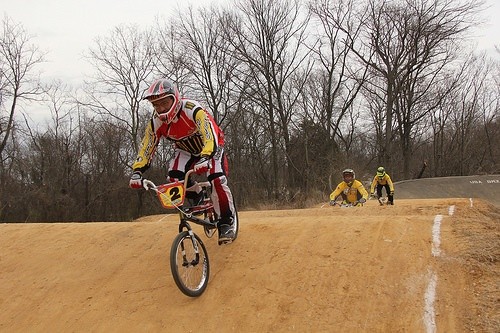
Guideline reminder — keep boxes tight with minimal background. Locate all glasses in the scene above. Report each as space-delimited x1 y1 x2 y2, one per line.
152 98 171 108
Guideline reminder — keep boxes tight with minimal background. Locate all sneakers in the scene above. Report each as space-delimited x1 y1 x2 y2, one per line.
216 216 235 245
192 193 205 216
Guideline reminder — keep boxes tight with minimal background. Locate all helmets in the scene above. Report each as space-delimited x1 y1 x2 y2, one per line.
143 79 181 124
377 167 385 176
342 169 355 180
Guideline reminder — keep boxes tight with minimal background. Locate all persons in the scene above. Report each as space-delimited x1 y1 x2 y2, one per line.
330 168 368 206
129 78 236 242
371 166 394 205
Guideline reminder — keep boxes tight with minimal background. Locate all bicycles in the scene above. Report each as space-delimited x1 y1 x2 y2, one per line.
334 201 363 207
129 162 239 297
370 193 394 206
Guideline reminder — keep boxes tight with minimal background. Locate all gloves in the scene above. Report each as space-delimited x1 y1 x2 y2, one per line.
370 193 375 197
330 200 336 206
359 198 366 203
390 191 394 195
130 171 144 188
194 157 212 175
